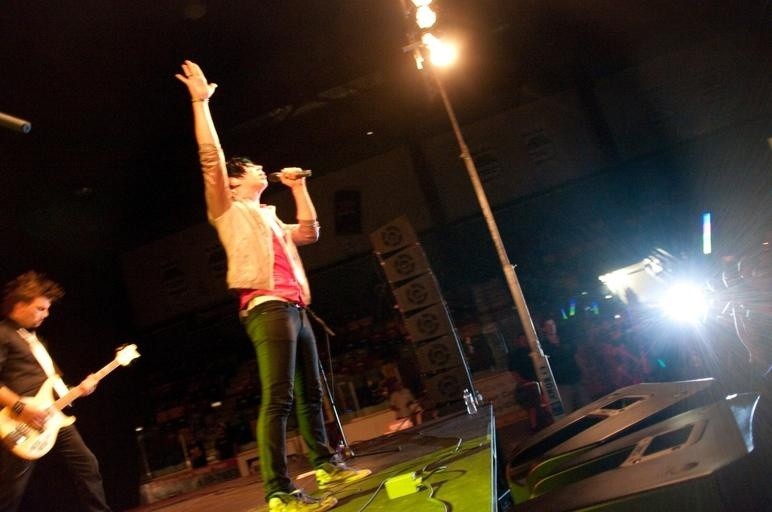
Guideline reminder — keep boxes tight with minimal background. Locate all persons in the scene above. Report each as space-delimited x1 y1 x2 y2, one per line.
176 58 372 511
0 268 118 508
507 238 771 512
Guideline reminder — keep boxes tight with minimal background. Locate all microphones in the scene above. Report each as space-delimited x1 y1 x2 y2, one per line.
267 170 313 183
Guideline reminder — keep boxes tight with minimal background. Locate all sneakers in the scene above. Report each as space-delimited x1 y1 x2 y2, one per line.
315 460 371 490
268 490 338 512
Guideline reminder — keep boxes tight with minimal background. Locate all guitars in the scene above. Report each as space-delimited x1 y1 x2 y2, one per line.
0 343 141 461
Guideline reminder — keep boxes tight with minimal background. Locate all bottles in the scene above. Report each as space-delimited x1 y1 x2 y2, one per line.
463 389 478 415
475 390 485 407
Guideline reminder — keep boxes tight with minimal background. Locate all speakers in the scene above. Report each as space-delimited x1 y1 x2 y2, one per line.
369 216 478 404
505 377 772 512
333 189 361 235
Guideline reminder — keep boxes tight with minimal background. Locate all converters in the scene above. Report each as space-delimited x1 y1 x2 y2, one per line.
385 472 420 500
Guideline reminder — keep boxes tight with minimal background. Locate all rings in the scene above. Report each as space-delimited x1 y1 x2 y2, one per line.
187 73 193 78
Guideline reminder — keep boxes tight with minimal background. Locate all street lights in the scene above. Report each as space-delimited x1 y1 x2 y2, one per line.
401 0 568 419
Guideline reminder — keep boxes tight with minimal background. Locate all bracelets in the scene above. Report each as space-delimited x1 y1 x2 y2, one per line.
12 400 25 416
191 98 210 104
